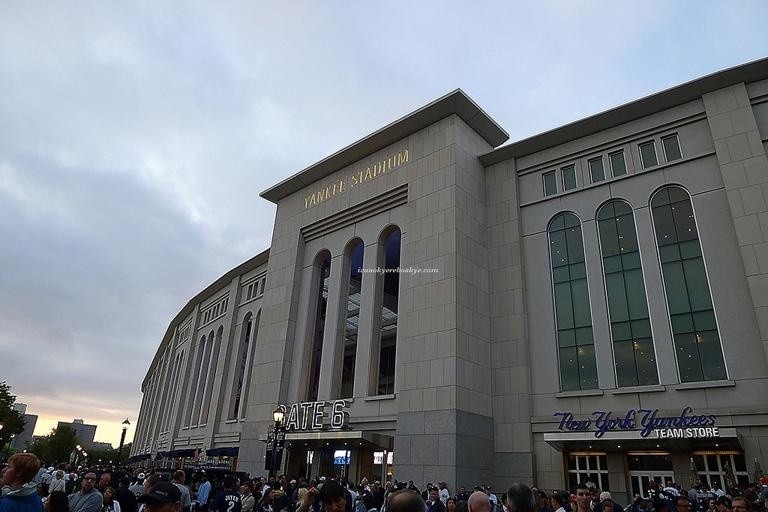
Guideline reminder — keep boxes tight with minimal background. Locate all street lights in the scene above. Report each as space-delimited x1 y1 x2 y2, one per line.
116 419 130 466
5 432 15 461
267 406 284 478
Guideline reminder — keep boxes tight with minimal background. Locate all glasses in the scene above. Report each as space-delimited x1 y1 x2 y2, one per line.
577 490 590 497
84 477 96 481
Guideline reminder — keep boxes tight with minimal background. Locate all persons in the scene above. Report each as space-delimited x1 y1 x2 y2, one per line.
0 447 767 511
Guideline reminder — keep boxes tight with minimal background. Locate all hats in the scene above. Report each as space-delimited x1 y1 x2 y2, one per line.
138 473 145 479
